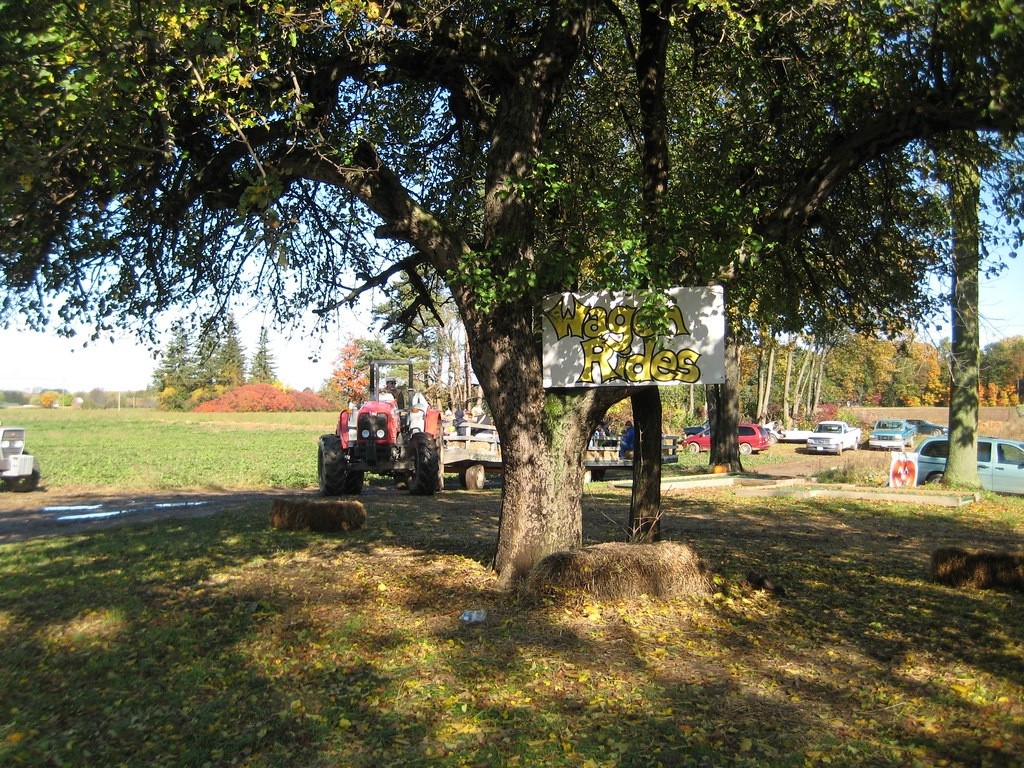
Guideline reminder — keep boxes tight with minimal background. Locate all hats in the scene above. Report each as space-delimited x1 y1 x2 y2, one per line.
386 377 396 382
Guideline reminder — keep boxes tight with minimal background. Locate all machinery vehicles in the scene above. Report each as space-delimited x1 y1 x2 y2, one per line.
0 426 41 494
318 355 680 499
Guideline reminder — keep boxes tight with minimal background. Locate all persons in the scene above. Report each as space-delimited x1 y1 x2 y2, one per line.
378 378 404 410
773 422 786 438
618 420 635 460
439 398 496 453
588 414 616 450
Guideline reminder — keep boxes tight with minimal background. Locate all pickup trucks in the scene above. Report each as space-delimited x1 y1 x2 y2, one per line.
806 420 862 456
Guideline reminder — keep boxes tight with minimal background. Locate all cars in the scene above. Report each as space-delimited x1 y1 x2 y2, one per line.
681 420 813 456
913 435 1024 497
906 419 949 437
869 418 918 452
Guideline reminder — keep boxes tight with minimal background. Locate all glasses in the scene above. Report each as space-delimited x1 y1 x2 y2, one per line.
391 381 396 385
